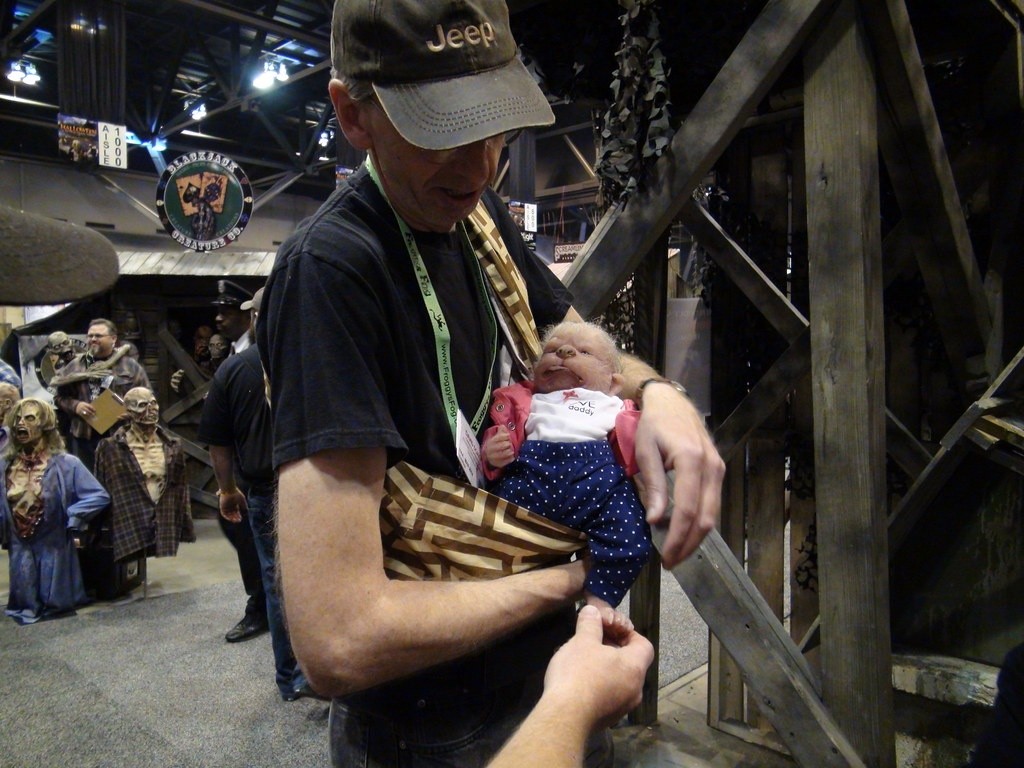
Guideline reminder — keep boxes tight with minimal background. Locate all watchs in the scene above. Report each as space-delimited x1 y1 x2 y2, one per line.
635 378 689 411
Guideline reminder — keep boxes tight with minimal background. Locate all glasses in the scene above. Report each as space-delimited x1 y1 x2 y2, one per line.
88 332 110 338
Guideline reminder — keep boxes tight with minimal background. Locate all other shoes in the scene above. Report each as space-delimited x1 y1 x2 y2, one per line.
281 682 333 701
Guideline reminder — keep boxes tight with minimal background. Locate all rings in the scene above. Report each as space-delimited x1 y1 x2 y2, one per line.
83 411 87 416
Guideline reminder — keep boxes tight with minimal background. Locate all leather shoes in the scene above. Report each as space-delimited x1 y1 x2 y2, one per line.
224 615 270 642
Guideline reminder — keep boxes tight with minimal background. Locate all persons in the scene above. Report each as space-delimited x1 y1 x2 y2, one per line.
261 0 727 767
54 317 153 477
195 285 305 703
483 605 655 768
204 280 270 643
481 321 652 643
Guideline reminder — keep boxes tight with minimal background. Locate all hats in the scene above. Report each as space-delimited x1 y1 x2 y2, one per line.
240 286 268 311
211 279 252 307
329 0 557 151
